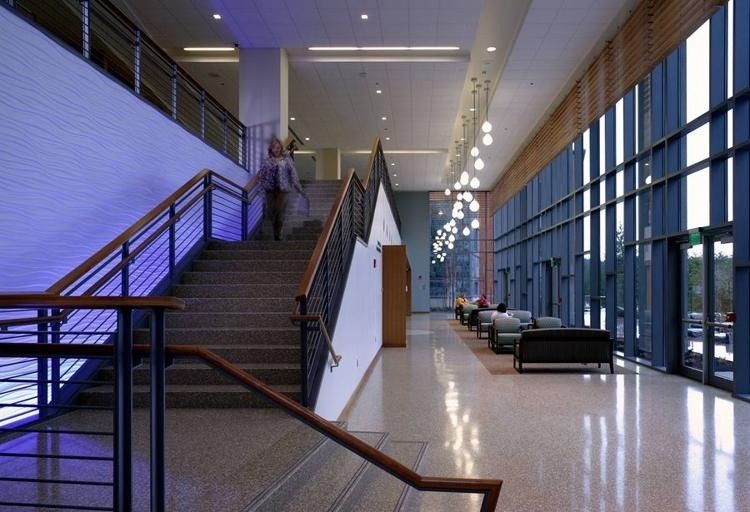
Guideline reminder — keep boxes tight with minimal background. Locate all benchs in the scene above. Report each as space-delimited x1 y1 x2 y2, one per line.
514 328 615 374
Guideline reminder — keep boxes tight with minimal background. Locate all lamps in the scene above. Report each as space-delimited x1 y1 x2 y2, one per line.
432 78 493 265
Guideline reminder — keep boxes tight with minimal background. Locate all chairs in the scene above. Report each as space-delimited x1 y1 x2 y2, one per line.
455 304 569 354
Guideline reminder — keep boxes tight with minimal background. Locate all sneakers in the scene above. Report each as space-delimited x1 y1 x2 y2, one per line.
274 233 283 241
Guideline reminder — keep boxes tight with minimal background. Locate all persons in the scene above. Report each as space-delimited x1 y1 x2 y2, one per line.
474 293 490 308
456 293 468 306
254 138 304 242
491 302 509 329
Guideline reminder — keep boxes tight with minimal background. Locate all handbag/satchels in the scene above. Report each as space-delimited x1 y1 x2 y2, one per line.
295 193 310 216
